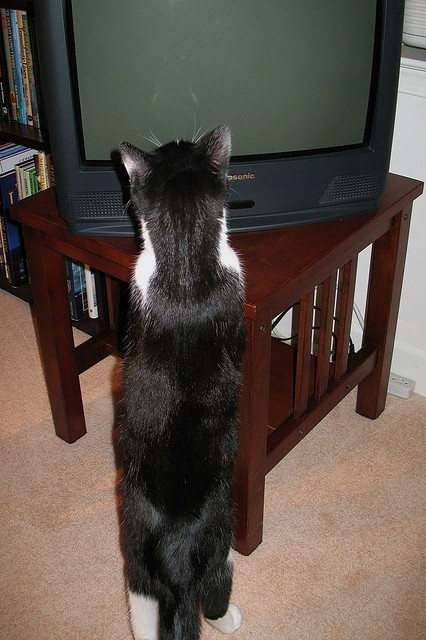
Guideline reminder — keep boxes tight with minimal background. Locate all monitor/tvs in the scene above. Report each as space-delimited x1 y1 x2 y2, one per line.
33 0 405 238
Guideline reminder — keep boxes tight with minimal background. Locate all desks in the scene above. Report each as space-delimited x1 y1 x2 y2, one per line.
7 172 424 556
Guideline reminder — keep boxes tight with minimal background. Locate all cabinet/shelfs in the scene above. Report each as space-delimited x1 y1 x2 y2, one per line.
0 0 106 337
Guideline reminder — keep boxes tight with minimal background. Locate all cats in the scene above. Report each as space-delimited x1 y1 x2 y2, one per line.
112 120 248 635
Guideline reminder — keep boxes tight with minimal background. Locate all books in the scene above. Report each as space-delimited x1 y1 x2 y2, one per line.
0 8 41 128
0 142 103 326
